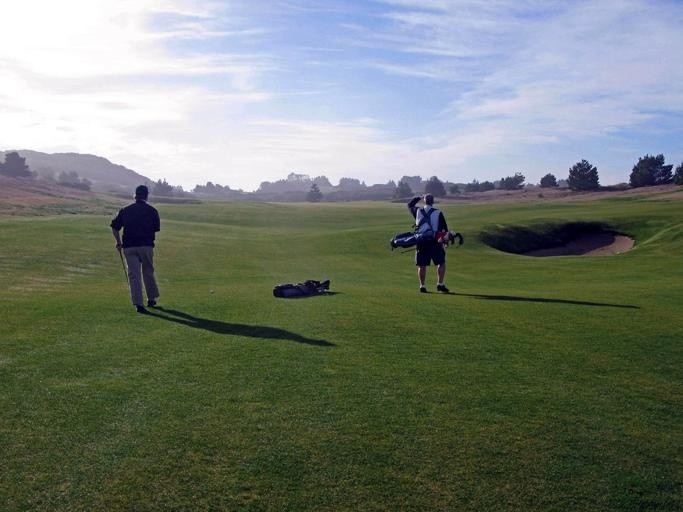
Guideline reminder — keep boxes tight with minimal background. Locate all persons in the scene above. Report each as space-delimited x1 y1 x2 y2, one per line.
406 192 450 293
110 184 160 312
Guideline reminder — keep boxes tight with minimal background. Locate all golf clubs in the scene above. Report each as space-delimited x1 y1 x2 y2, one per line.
120 251 130 289
400 233 464 254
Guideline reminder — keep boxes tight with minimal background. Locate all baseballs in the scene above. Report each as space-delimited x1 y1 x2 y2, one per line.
211 290 215 294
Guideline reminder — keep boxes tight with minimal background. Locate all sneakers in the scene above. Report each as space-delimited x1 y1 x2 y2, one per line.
436 284 448 293
136 305 146 313
146 299 156 306
419 286 427 292
